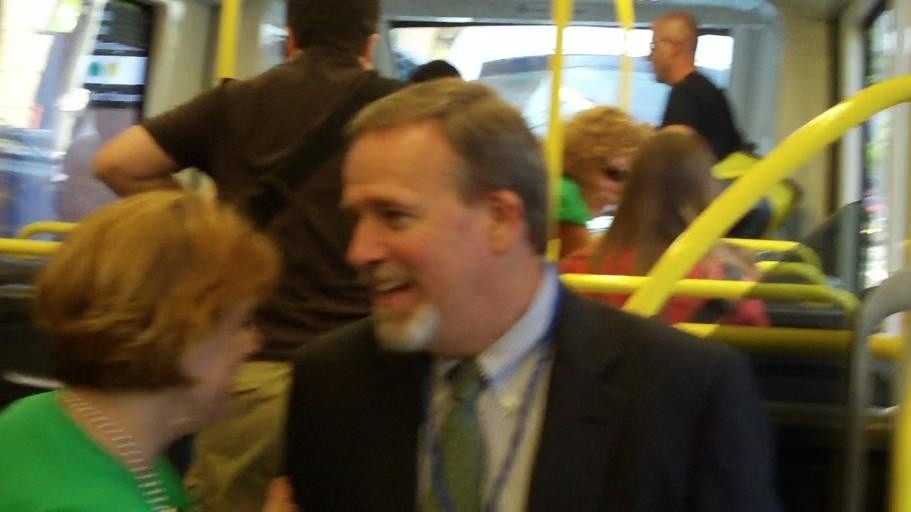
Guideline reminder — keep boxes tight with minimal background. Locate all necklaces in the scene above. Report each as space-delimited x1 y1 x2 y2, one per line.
62 386 171 512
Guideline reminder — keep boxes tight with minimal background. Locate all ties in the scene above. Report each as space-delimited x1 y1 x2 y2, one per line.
431 357 491 510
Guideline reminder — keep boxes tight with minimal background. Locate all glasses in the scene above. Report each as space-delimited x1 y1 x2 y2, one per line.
601 166 629 183
649 38 673 53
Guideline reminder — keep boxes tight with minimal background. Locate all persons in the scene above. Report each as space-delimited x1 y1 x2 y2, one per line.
98 1 420 512
411 59 461 82
556 105 654 257
645 11 771 238
554 124 770 327
0 187 284 512
284 75 776 512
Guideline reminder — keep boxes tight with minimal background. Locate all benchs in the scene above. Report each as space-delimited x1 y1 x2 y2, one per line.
560 238 911 512
0 218 78 391
